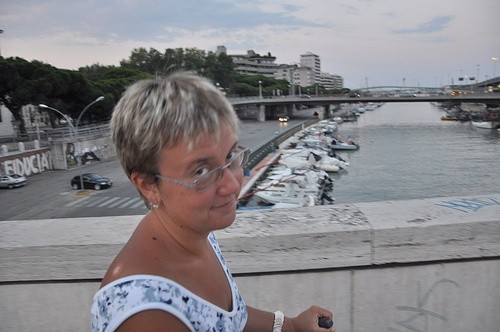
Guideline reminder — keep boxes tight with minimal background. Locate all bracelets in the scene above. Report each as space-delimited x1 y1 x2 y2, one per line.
272 310 284 332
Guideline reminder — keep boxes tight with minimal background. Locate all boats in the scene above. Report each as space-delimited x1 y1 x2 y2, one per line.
254 101 371 210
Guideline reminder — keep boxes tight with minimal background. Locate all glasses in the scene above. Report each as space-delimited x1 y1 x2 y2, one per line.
152 144 252 192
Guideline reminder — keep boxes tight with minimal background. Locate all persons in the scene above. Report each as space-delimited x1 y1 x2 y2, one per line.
89 73 335 332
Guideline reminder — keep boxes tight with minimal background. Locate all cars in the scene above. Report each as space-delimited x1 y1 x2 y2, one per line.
0 173 27 190
71 172 113 191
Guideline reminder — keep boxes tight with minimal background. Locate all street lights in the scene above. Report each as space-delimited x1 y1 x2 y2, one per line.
258 80 263 100
39 96 106 192
